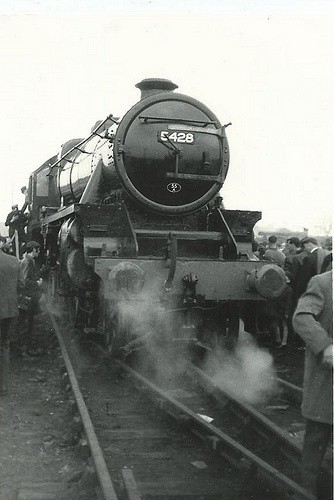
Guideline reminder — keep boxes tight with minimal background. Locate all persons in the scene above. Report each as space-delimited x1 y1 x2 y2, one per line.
252 235 333 491
0 187 45 396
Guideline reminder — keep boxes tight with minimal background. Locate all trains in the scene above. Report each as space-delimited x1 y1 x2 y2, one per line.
4 77 290 359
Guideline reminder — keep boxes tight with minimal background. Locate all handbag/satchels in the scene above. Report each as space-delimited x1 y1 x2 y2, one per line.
17 295 33 313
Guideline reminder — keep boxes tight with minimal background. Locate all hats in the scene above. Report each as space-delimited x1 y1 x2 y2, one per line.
26 240 40 250
300 237 317 246
